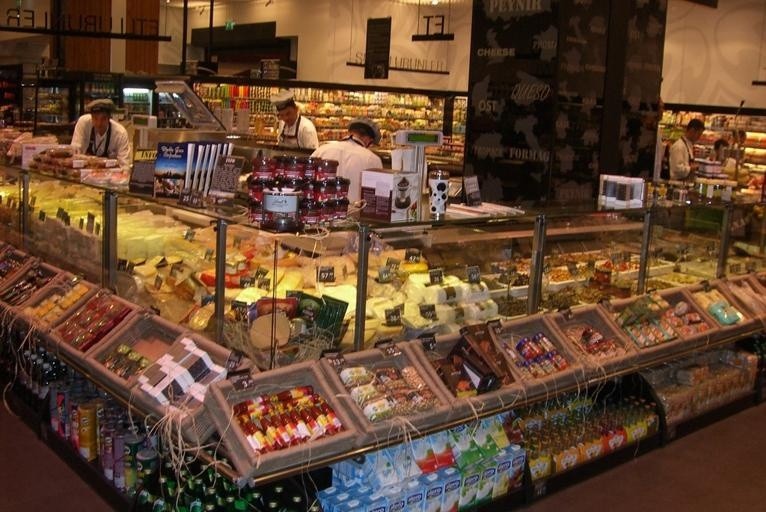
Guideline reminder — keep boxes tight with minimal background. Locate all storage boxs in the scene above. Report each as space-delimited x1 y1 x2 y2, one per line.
361 169 425 224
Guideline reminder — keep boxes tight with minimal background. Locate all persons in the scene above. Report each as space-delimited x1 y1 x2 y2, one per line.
308 114 382 206
269 92 319 150
668 119 705 180
70 99 131 177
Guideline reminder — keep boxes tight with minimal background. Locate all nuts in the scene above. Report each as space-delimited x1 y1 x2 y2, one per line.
377 366 439 415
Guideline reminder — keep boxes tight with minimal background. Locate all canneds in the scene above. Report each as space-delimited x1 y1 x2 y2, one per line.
133 93 148 101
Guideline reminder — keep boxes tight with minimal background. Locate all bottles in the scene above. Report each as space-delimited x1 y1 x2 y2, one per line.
135 449 318 512
524 393 659 482
233 385 344 453
15 346 67 429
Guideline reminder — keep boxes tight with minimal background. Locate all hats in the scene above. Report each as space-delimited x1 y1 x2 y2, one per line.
269 91 294 109
86 98 113 113
349 116 381 145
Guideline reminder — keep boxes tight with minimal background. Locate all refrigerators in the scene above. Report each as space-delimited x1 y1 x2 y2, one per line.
72 69 194 130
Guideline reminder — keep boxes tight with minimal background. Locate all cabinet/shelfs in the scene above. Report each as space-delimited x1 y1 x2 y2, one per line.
200 84 468 171
21 77 193 141
0 161 766 512
659 103 766 203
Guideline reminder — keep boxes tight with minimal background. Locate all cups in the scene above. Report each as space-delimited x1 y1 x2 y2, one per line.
429 170 449 219
